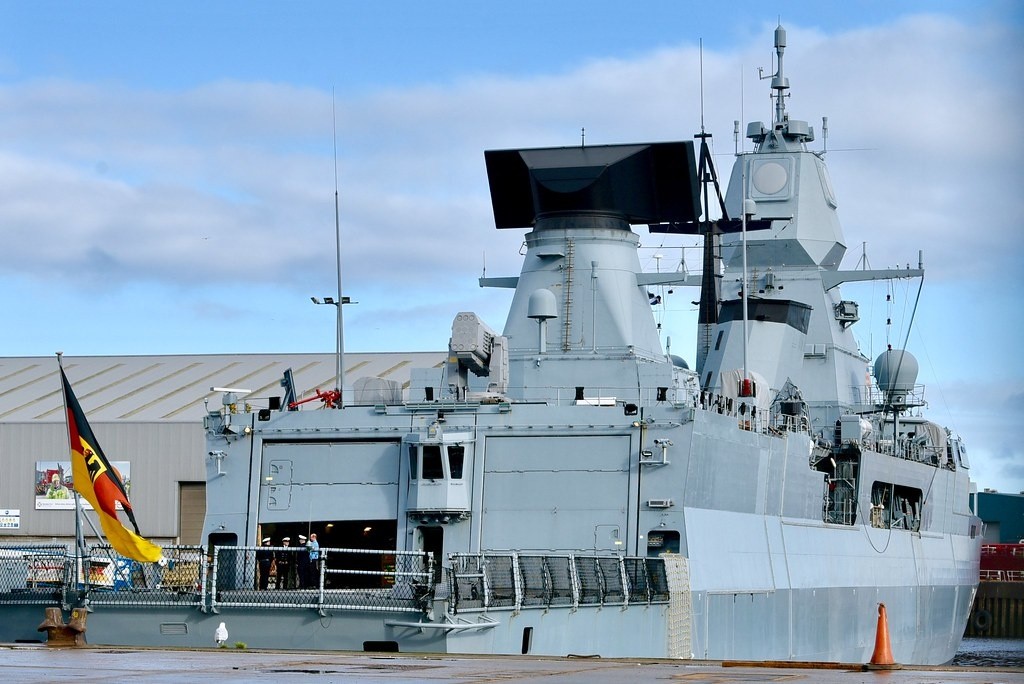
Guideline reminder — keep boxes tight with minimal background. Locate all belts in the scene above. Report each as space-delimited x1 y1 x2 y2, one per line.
259 558 271 562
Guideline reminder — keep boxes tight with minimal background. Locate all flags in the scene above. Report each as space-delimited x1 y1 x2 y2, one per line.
59 365 161 562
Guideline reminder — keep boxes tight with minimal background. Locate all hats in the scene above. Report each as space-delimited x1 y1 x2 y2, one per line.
51 474 60 483
282 537 290 542
261 537 271 543
297 535 307 540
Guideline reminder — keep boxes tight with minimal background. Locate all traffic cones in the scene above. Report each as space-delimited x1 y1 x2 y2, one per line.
863 604 903 669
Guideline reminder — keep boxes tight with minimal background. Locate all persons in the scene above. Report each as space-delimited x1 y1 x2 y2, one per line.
274 537 292 591
257 537 273 590
308 533 320 586
46 474 70 499
296 534 310 589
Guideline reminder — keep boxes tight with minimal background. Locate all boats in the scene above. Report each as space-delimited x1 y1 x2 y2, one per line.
0 15 985 665
961 488 1024 640
